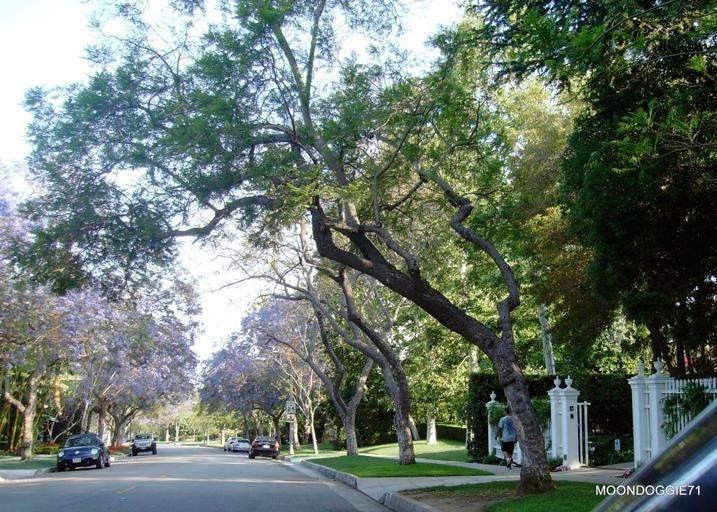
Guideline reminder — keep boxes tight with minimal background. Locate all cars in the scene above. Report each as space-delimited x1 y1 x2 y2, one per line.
249 436 280 459
223 436 250 453
57 433 110 471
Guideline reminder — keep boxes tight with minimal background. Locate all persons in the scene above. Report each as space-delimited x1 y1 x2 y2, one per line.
495 407 517 470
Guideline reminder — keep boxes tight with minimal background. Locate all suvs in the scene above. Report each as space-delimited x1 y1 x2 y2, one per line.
132 433 157 456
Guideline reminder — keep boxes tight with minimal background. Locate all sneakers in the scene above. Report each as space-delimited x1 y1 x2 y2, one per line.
506 457 513 469
